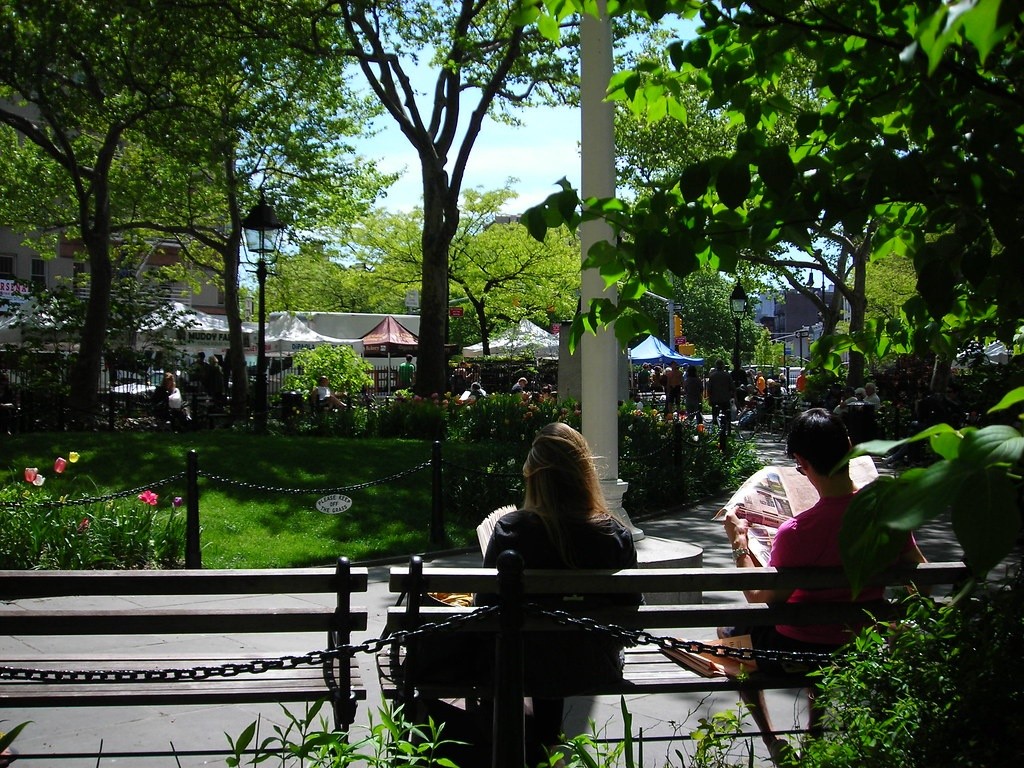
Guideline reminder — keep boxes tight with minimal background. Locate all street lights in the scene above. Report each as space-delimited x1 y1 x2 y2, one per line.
238 190 288 438
806 258 827 306
728 278 749 369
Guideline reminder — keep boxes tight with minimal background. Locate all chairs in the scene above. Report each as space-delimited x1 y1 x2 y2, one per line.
308 393 351 415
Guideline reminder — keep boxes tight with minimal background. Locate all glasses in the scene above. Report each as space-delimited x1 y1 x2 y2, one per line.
796 462 806 476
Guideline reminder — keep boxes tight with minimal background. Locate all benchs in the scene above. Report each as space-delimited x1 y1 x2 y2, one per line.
179 392 229 417
743 392 810 430
373 558 972 768
0 552 369 768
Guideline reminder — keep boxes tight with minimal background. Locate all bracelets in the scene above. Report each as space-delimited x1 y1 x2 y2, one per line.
733 548 751 558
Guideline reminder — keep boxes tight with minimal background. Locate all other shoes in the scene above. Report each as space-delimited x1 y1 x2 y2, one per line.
771 740 801 768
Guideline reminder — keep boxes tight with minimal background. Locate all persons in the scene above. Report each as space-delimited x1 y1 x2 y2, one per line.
157 353 992 768
475 424 641 768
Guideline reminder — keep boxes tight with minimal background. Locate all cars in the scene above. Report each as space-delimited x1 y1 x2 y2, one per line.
110 368 188 397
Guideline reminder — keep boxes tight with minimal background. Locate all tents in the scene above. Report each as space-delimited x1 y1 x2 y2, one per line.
0 301 704 396
358 315 419 395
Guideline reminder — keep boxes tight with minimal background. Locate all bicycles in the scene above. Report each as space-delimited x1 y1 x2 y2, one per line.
737 392 789 444
